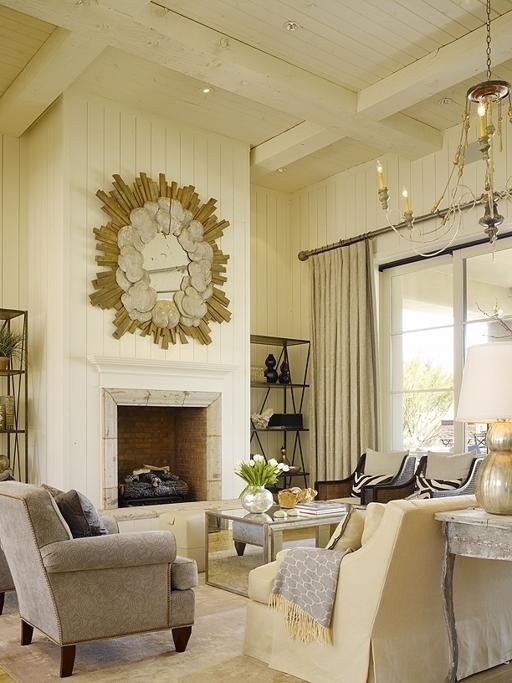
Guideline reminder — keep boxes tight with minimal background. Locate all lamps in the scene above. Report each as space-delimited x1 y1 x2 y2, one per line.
375 0 512 258
456 341 511 516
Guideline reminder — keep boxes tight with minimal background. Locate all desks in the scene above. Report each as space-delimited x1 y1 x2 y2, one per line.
433 508 511 682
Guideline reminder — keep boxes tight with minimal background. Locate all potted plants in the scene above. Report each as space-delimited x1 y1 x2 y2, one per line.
1 321 31 371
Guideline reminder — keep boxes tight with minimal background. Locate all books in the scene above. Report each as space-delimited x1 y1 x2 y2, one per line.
298 506 347 514
295 501 345 510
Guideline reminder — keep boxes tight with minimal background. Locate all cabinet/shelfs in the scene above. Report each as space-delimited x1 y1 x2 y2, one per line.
250 334 311 492
0 308 27 482
